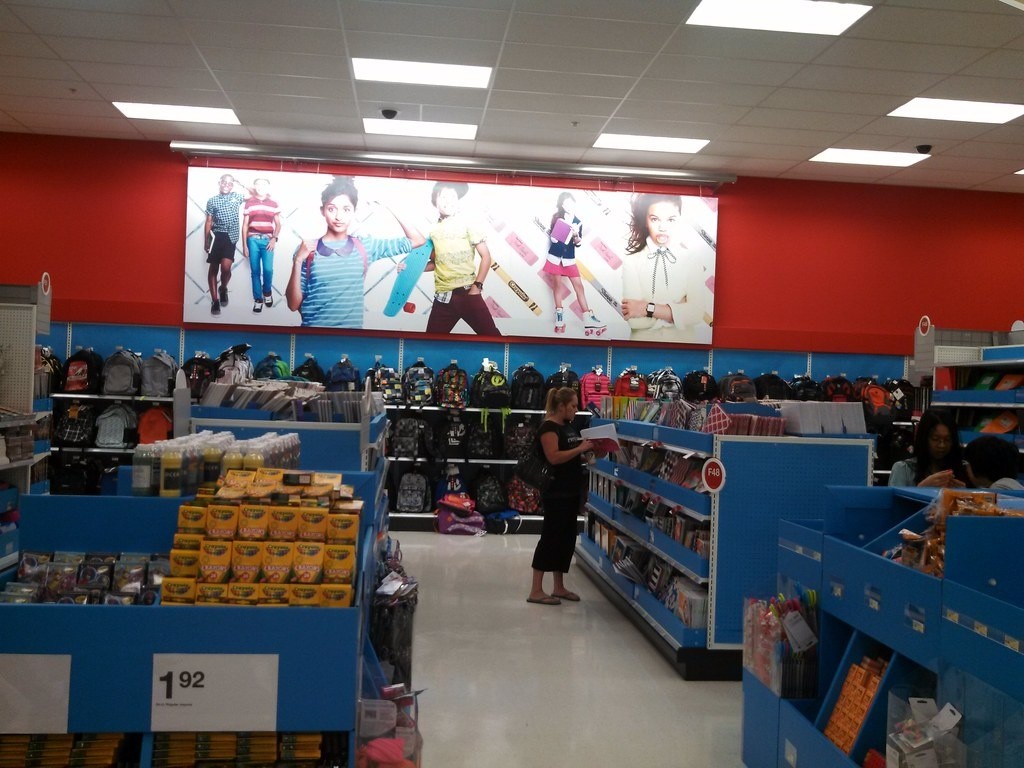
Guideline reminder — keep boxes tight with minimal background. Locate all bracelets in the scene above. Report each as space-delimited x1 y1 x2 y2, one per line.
270 236 278 242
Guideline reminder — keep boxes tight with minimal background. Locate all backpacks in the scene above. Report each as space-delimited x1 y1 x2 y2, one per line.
34 344 915 536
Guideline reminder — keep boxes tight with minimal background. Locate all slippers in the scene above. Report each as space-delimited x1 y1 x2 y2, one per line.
527 595 561 604
551 593 580 601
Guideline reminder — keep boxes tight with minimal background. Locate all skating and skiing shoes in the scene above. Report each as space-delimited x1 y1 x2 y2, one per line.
554 307 566 333
583 309 607 336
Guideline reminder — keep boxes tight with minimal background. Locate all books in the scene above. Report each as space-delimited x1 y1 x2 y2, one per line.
579 423 622 453
206 230 215 254
584 394 788 628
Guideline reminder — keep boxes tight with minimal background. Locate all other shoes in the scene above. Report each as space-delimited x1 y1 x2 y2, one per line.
264 291 272 307
253 299 263 312
218 287 230 307
211 300 221 318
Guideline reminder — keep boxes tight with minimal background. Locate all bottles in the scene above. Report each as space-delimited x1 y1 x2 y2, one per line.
128 429 301 499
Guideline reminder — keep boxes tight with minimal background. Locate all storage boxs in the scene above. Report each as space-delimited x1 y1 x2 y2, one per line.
592 472 644 567
162 468 365 608
0 733 322 768
824 656 889 753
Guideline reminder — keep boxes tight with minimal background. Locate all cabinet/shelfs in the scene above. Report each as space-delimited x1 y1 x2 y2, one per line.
930 344 1024 453
0 419 31 591
741 486 1024 768
0 468 380 768
575 418 877 679
31 412 53 466
48 393 594 521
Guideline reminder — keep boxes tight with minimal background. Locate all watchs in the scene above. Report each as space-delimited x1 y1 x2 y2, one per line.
474 281 484 289
646 302 655 318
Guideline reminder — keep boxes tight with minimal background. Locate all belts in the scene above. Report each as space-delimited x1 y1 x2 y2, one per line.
253 234 272 240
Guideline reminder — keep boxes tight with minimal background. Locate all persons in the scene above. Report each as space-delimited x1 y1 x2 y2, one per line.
242 174 282 313
543 191 608 329
887 408 980 490
526 385 608 607
965 435 1024 491
286 175 427 330
204 174 256 315
620 193 705 344
397 180 502 337
